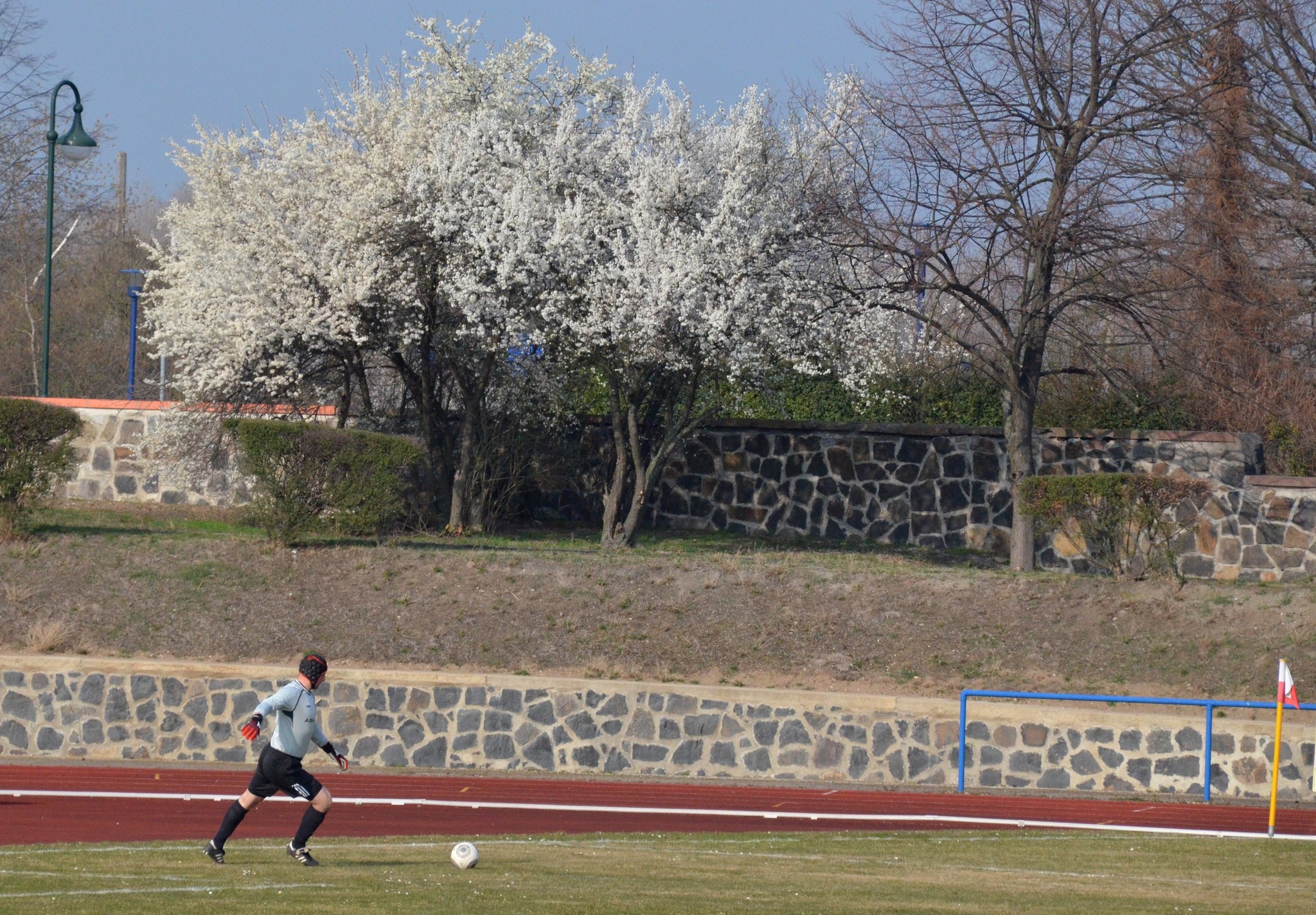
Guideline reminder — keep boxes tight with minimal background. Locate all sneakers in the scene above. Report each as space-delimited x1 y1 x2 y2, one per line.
287 838 319 866
201 839 224 863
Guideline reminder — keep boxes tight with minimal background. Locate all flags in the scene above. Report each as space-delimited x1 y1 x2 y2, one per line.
1276 661 1300 709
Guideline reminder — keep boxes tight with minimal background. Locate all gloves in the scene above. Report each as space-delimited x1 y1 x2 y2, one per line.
240 713 263 741
323 745 348 771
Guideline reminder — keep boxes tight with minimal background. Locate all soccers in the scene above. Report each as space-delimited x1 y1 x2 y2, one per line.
450 842 478 870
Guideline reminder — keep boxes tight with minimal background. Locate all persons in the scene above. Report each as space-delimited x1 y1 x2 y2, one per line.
203 654 349 866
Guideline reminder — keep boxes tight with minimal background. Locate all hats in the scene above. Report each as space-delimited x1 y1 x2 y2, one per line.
298 654 328 685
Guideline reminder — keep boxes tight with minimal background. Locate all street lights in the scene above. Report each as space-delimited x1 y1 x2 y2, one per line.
117 266 148 398
912 220 936 347
41 79 98 400
